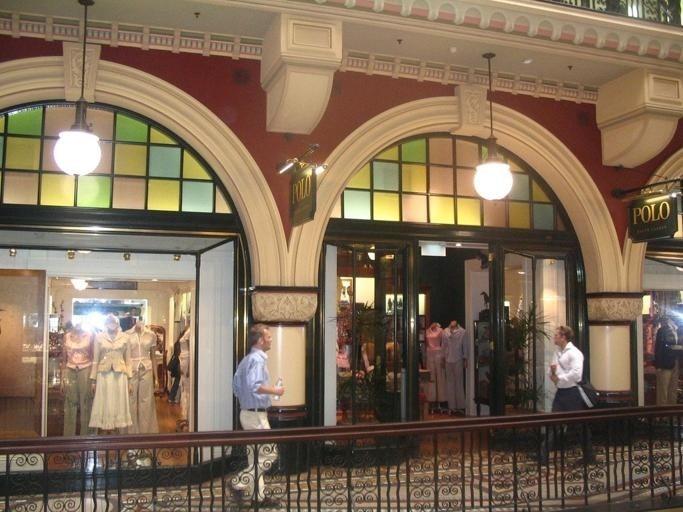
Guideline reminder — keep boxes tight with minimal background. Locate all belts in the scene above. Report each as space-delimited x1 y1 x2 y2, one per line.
557 385 578 391
240 406 268 414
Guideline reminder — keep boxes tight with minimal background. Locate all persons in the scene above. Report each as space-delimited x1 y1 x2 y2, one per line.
655 318 683 423
527 326 596 466
60 310 190 465
441 320 468 410
425 323 443 402
227 323 285 508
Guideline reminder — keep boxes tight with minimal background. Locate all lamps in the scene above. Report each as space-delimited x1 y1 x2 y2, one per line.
174 254 182 260
9 249 16 255
68 250 75 259
474 53 514 201
50 1 102 178
124 254 130 260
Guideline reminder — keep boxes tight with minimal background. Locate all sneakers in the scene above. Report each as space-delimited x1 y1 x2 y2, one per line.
249 496 281 507
577 451 595 465
167 399 179 403
227 480 251 507
525 451 549 466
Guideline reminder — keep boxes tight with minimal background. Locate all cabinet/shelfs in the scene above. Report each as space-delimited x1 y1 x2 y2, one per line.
32 352 81 437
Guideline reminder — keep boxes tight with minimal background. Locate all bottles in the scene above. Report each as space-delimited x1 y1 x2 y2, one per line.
272 377 284 401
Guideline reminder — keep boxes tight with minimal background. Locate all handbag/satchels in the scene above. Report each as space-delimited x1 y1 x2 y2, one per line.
166 355 179 377
577 376 599 409
